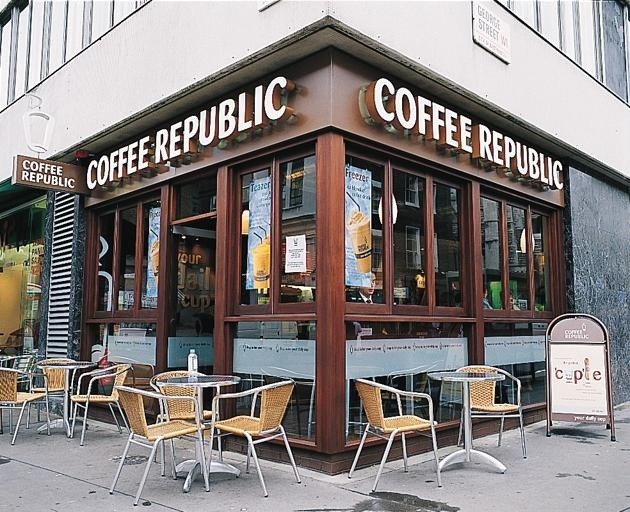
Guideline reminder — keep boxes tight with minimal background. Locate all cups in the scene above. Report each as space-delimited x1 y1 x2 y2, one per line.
346 218 372 273
251 248 270 288
150 248 159 285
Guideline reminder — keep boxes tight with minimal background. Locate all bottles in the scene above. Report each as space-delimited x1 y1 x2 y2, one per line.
187 348 198 378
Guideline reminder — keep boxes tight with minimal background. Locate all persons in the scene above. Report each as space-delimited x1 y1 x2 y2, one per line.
499 289 520 311
346 271 391 335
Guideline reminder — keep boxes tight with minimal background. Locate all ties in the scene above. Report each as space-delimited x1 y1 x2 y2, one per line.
367 299 372 304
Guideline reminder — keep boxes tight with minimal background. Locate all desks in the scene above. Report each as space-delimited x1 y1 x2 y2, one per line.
36 362 95 438
158 375 241 493
428 372 506 473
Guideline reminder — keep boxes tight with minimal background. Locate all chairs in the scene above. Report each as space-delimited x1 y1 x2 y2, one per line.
69 363 130 445
455 365 528 460
110 385 210 505
349 378 443 491
12 355 34 393
151 370 209 481
37 358 75 429
0 368 50 445
206 378 302 497
